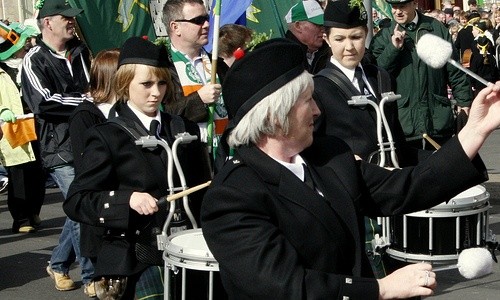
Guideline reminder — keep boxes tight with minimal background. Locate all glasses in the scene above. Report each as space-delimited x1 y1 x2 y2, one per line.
175 14 209 25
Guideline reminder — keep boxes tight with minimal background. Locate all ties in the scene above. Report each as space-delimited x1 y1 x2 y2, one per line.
302 163 315 191
354 67 372 95
148 120 161 140
405 22 416 31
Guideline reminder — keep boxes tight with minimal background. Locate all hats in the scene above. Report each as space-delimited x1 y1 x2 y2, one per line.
473 21 486 32
117 37 169 70
34 0 84 19
9 22 36 37
478 36 489 64
465 13 480 23
444 8 454 14
0 21 27 60
324 0 367 29
222 38 306 129
285 0 324 25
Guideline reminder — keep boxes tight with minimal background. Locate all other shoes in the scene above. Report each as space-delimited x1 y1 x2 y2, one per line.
19 226 36 233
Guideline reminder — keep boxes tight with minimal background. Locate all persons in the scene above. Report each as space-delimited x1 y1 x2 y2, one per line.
61 37 212 300
323 0 437 280
161 0 234 163
21 0 97 297
369 0 473 138
284 0 333 75
431 0 500 95
0 22 48 232
218 24 252 60
69 50 121 257
198 38 500 300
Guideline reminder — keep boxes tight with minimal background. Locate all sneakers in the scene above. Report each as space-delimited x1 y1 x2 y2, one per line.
84 282 96 297
46 265 76 291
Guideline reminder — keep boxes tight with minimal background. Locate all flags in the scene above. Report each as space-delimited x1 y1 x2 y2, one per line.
69 0 299 54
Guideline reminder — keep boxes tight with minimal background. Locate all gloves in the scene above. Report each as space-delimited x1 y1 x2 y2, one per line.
0 108 16 123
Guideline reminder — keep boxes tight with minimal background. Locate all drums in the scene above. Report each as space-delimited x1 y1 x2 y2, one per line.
375 179 491 267
160 228 231 300
